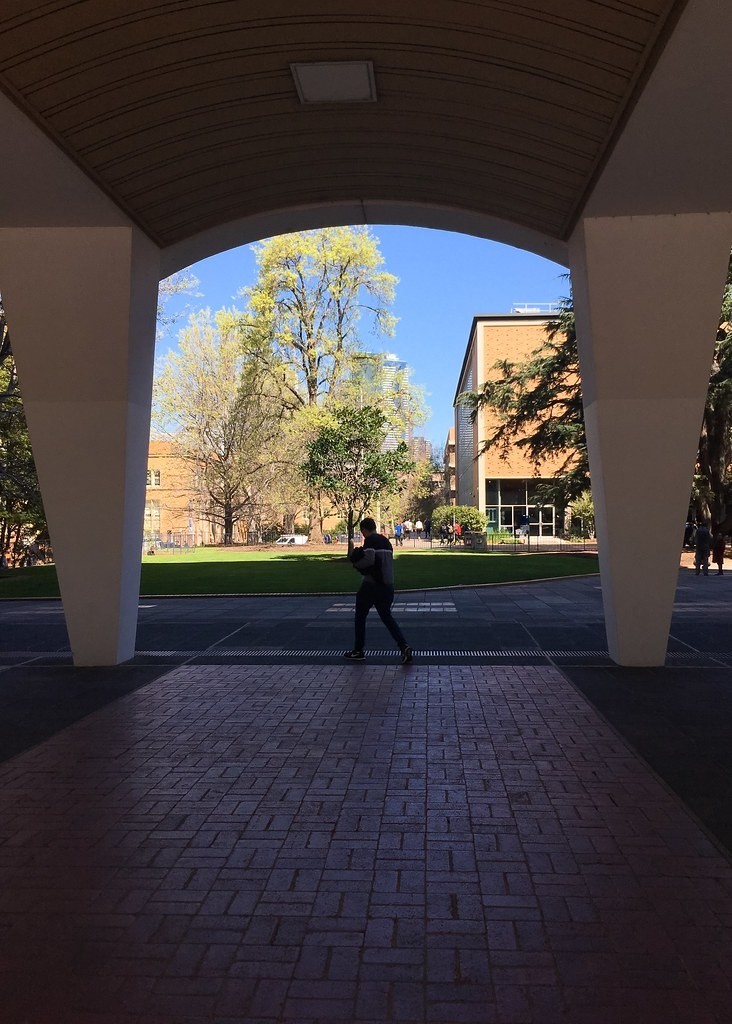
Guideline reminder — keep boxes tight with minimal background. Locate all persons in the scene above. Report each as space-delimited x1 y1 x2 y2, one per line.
343 518 413 664
402 518 431 540
183 541 188 548
712 534 725 575
395 522 403 546
682 521 732 553
438 520 469 545
695 521 711 576
323 530 342 544
355 530 365 545
175 541 181 548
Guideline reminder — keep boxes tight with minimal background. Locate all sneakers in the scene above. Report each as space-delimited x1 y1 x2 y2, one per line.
400 644 412 664
344 651 365 660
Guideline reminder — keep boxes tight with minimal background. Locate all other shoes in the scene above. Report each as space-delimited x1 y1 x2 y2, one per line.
714 570 723 575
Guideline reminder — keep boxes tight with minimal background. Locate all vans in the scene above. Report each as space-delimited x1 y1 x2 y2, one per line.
278 534 308 546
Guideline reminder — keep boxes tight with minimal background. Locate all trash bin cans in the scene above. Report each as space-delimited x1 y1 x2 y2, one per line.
463 530 487 549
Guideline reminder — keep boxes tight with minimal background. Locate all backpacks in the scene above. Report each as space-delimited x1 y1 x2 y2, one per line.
447 525 453 533
